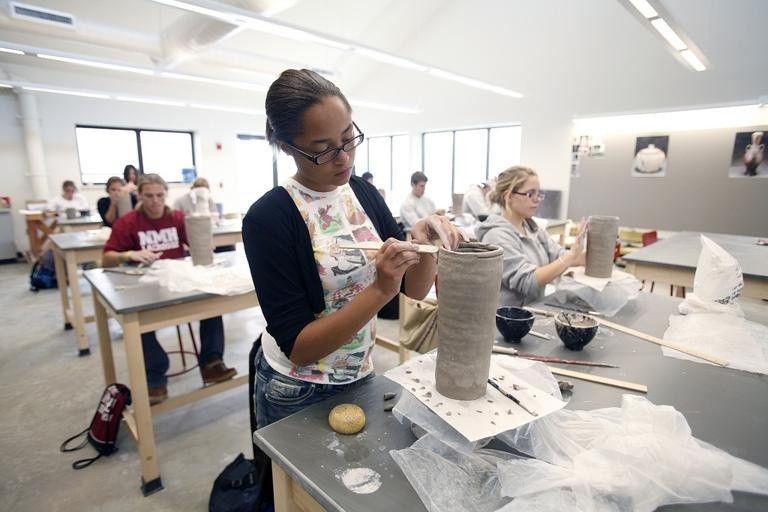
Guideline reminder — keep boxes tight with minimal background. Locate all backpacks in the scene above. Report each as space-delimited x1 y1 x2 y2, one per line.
28 249 60 294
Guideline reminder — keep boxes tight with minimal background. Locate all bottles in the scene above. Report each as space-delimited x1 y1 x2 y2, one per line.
635 143 665 173
743 132 765 176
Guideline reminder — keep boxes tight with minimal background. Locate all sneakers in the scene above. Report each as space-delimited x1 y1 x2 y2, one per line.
199 361 237 386
147 385 171 406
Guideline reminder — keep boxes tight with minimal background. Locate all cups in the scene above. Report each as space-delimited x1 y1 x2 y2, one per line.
584 213 620 280
451 194 465 217
184 214 218 268
434 241 501 402
115 189 131 219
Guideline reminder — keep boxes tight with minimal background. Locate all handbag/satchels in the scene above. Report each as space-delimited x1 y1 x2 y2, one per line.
60 383 134 471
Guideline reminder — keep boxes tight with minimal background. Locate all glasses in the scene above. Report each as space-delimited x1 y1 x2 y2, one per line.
511 190 547 202
280 118 366 166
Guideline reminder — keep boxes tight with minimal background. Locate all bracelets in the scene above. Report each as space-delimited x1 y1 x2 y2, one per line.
558 255 570 269
116 251 133 263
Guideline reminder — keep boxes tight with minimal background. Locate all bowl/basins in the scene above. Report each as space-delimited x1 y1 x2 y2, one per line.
554 310 600 352
496 305 536 345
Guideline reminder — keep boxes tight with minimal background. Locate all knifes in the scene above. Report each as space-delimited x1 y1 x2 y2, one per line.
340 242 438 254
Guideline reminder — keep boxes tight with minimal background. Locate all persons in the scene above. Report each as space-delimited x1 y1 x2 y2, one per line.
173 176 235 255
244 66 472 512
103 173 238 407
123 166 140 183
363 172 376 187
473 165 588 313
97 176 138 230
400 167 445 235
43 182 97 271
